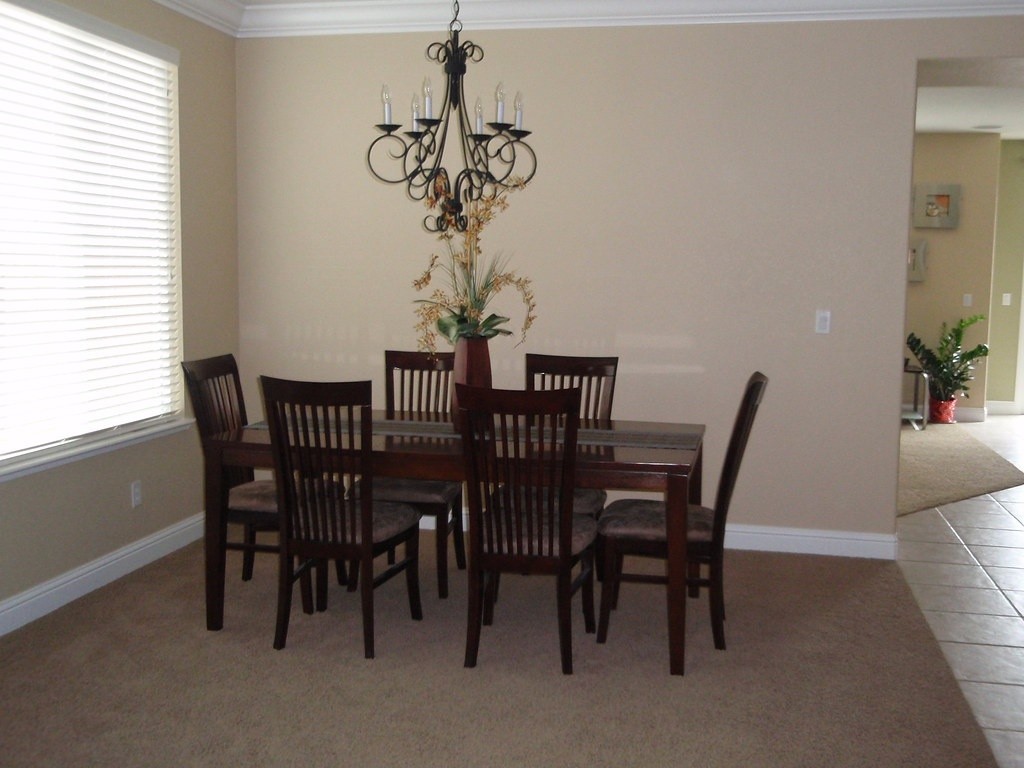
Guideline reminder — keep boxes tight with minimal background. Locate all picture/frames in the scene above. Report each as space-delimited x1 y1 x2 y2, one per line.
912 182 962 229
908 239 928 281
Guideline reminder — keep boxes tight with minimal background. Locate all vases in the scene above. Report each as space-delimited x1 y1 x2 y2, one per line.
452 336 492 418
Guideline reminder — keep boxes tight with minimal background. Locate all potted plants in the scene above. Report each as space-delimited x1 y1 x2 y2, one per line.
907 316 989 424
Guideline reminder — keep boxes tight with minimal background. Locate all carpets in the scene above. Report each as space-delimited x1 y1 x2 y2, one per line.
897 424 1024 518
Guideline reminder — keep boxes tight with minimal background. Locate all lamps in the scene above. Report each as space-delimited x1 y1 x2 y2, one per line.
367 0 537 231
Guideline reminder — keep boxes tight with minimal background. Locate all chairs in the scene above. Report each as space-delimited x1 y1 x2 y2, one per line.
595 370 768 649
347 351 466 597
485 353 619 580
181 355 347 615
256 376 423 659
456 383 596 676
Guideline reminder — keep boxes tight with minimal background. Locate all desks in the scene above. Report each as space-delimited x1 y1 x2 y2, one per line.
901 365 929 432
212 403 706 676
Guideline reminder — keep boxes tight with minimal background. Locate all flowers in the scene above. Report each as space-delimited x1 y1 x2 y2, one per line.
411 169 535 359
